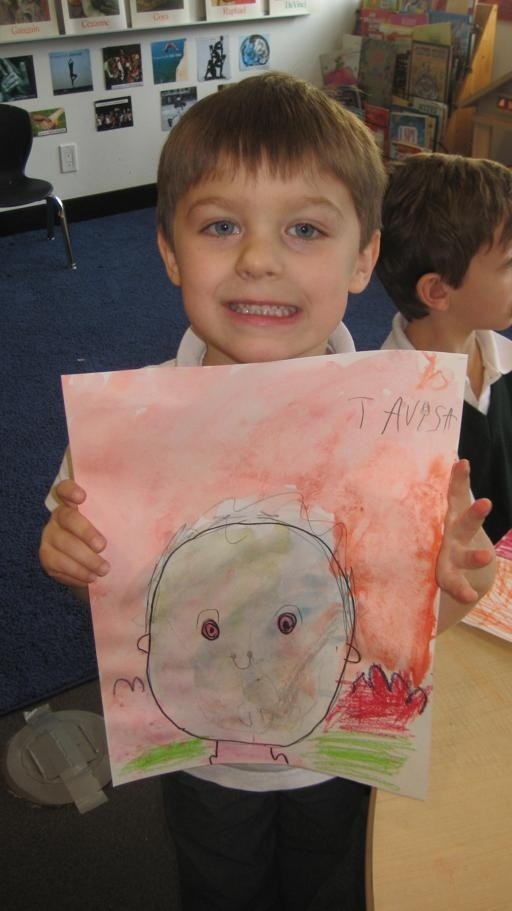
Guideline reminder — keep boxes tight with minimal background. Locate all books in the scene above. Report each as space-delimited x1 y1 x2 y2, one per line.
317 0 481 160
0 1 313 43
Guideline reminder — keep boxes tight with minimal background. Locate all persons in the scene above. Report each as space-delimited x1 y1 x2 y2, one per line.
203 35 226 80
167 96 187 128
65 55 77 86
0 57 34 103
31 109 65 131
103 47 144 90
374 152 512 543
96 105 133 132
158 42 180 54
37 70 498 910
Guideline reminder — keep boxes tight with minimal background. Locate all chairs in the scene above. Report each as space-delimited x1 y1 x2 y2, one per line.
0 101 79 274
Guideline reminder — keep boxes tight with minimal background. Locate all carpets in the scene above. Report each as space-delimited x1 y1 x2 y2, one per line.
2 208 394 720
